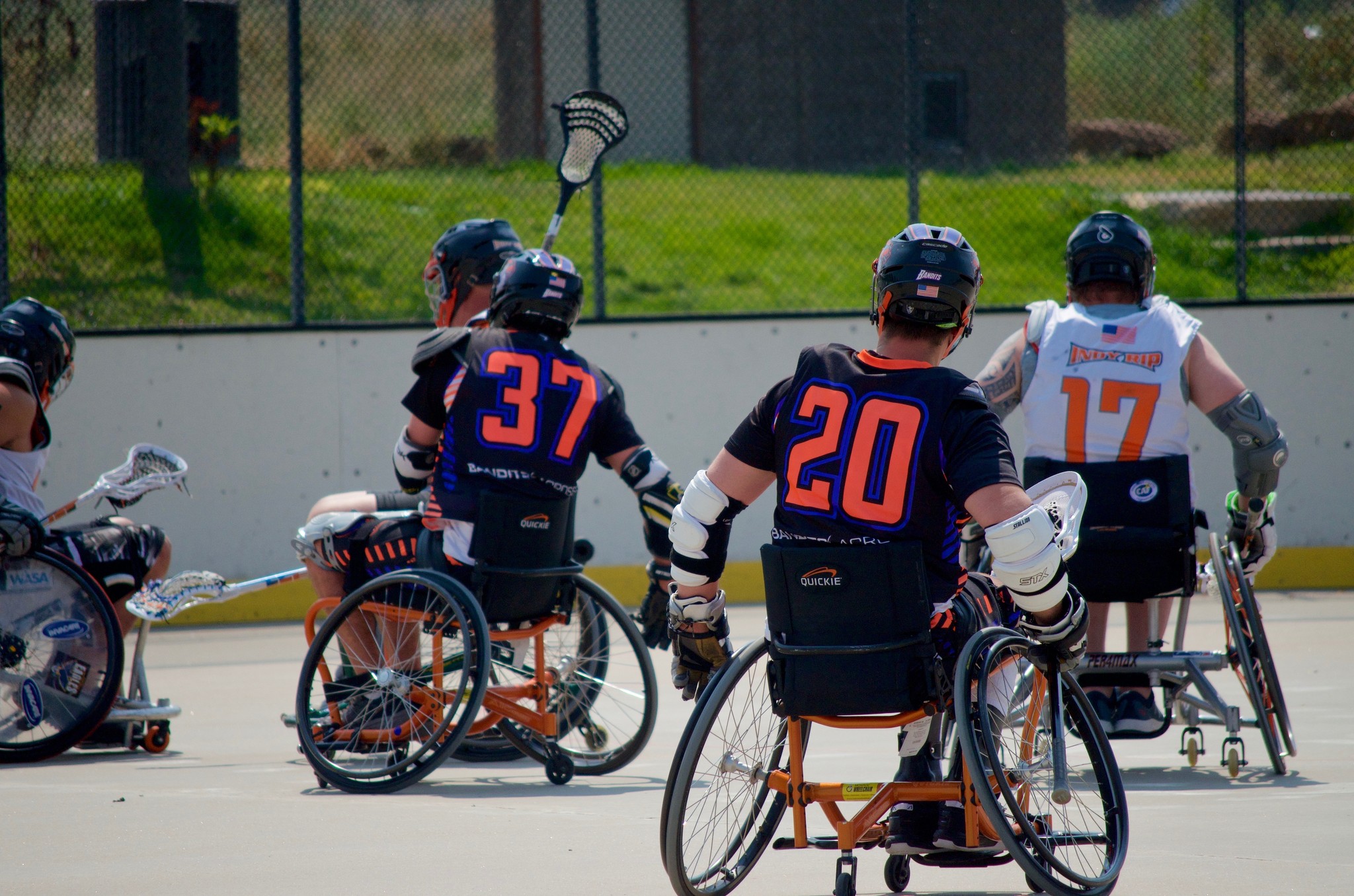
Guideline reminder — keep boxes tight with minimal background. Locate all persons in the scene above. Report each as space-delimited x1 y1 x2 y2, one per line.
0 296 172 752
664 223 1089 852
297 220 681 735
958 209 1288 738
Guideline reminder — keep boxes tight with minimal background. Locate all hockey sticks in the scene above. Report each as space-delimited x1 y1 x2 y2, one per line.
541 89 629 254
1204 496 1265 605
1026 471 1088 804
38 442 187 526
125 567 309 622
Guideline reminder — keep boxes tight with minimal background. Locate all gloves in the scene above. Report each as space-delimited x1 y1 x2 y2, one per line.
641 580 672 652
666 582 734 704
1018 582 1090 673
0 493 46 558
1224 490 1277 586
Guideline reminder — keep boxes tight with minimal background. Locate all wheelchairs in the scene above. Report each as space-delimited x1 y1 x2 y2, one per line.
998 453 1299 778
657 508 1133 896
0 488 183 767
279 492 659 797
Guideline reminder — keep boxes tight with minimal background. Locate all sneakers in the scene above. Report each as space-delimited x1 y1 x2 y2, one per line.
885 800 948 854
932 804 1006 854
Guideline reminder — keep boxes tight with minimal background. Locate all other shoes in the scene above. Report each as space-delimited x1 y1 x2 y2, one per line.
13 670 137 743
1115 691 1164 735
343 690 433 738
1084 690 1115 736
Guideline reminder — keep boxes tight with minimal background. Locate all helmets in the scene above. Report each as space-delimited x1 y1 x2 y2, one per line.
871 221 983 359
424 220 522 328
484 247 584 339
0 296 74 412
1064 210 1156 304
